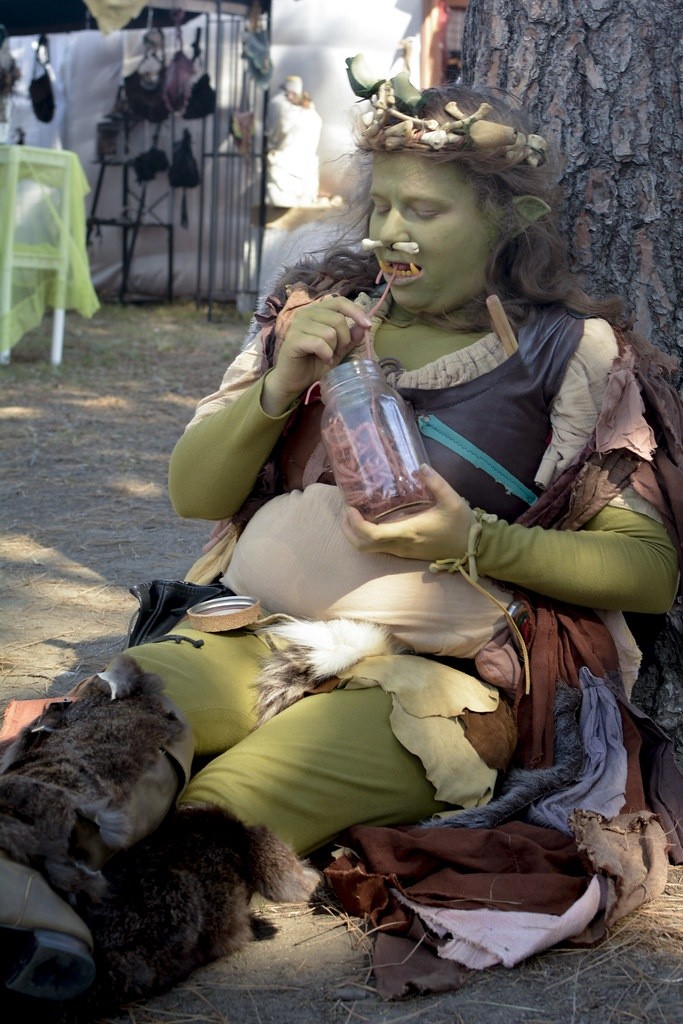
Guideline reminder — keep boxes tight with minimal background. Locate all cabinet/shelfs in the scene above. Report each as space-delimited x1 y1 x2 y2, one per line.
0 146 78 366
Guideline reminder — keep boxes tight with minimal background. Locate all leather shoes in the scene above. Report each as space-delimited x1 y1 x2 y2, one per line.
0 857 96 1001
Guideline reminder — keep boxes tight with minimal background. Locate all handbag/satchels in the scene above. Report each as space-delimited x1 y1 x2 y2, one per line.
133 145 168 186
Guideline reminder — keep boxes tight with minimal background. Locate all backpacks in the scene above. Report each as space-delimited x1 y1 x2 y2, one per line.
182 74 217 118
168 129 199 229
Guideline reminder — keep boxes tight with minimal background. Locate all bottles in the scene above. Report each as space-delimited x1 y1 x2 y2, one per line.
318 360 436 526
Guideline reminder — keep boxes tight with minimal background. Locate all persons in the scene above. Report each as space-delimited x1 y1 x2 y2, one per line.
0 79 683 1024
266 75 322 203
